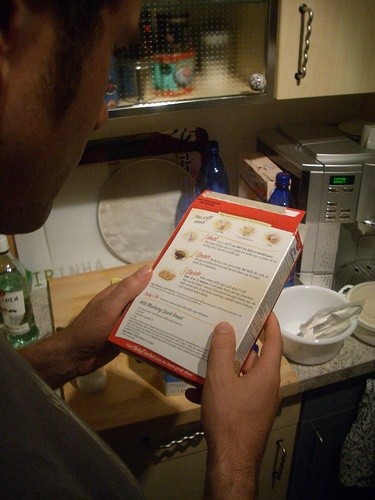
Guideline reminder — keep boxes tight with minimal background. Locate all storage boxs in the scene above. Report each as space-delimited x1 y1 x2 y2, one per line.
237 152 291 202
162 373 189 397
107 188 308 387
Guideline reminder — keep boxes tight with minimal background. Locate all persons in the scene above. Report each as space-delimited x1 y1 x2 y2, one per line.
0 0 282 500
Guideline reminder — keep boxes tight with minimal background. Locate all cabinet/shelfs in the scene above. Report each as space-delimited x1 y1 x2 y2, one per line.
108 0 375 119
105 372 365 500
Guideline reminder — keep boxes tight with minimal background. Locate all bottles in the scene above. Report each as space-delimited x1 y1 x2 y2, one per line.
0 233 41 350
77 366 108 394
267 172 295 288
199 140 229 197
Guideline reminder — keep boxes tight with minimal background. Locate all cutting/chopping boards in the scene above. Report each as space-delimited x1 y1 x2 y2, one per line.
46 260 304 431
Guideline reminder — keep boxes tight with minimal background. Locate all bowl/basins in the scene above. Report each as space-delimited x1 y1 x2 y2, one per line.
337 281 375 346
272 285 359 365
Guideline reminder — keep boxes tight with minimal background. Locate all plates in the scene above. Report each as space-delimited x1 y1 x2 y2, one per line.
97 158 198 264
